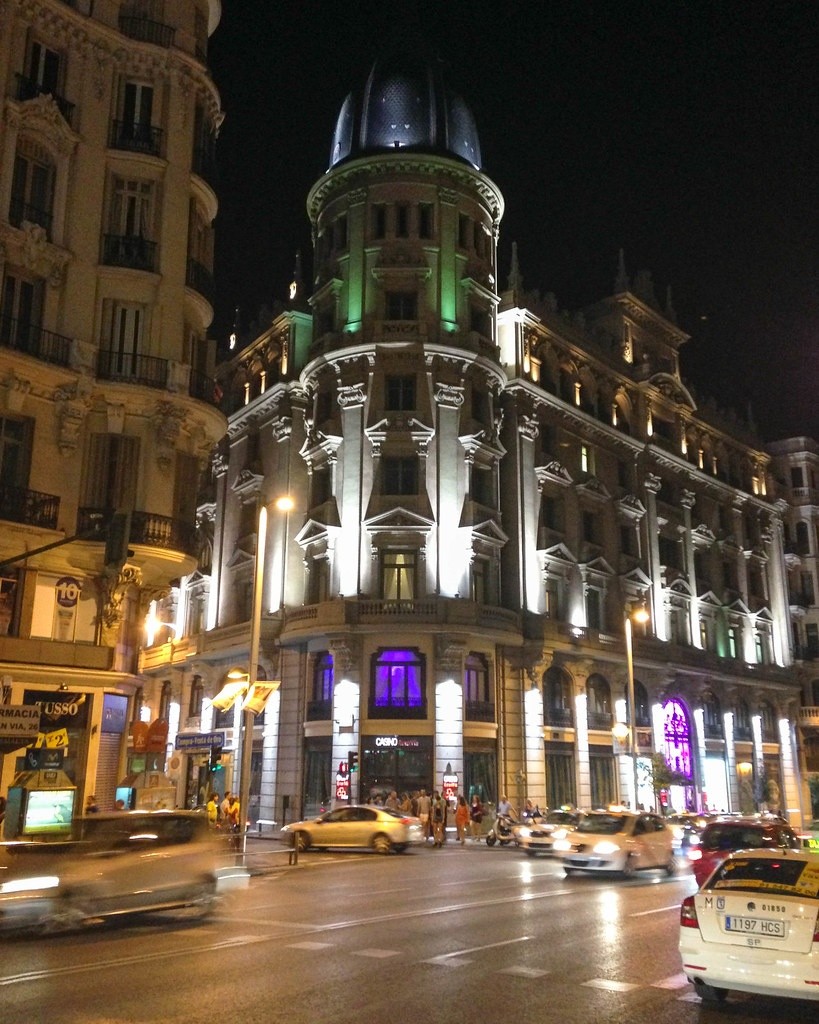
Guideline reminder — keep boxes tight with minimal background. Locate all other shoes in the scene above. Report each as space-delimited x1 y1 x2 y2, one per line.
456 837 460 840
461 840 465 846
499 842 503 846
476 838 480 841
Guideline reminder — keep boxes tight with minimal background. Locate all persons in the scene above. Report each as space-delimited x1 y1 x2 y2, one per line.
759 779 781 817
113 799 125 810
85 795 98 814
206 791 250 852
366 789 537 848
618 801 726 814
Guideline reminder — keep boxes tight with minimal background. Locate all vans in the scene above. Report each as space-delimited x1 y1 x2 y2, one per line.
0 808 222 931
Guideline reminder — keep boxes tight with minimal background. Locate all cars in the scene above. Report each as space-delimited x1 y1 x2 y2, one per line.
519 808 580 857
678 847 819 1002
689 815 810 890
554 810 679 880
278 804 426 855
666 810 746 861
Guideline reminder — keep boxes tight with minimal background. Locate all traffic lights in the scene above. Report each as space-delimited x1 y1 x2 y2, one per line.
349 751 359 773
103 510 138 569
210 745 222 773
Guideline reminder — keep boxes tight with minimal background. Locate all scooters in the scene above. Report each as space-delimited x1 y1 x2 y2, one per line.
485 812 531 847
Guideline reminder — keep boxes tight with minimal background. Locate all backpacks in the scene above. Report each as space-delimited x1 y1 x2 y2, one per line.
434 805 443 822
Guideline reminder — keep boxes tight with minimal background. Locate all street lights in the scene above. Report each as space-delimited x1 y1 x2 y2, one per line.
237 493 295 850
624 607 649 808
